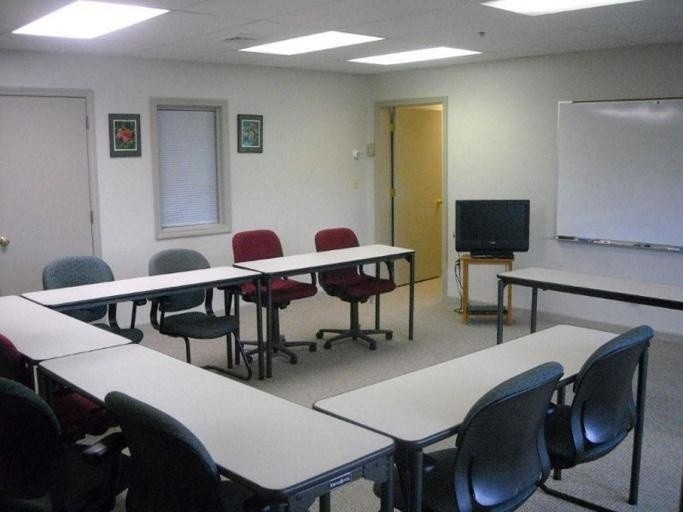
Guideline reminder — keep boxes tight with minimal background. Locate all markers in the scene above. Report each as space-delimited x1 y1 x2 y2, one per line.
667 248 681 252
576 239 611 244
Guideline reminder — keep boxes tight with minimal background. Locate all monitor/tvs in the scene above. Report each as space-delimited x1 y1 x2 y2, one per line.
455 200 529 259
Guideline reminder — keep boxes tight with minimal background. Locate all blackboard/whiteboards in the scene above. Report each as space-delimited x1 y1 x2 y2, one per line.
554 97 683 251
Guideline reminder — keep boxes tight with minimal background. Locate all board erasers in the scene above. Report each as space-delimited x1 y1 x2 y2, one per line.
559 236 574 240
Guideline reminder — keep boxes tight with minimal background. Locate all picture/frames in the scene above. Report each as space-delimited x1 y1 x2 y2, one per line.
237 113 263 153
108 113 141 158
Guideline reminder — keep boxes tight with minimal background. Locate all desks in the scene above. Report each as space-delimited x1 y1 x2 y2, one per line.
38 344 395 509
20 266 265 381
460 255 516 324
313 324 652 508
0 295 132 408
234 244 416 377
489 264 682 344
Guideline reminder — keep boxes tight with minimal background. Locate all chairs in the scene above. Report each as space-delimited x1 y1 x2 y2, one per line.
106 392 267 509
149 248 253 380
315 229 398 351
3 337 99 436
43 255 144 344
545 325 654 481
233 230 318 364
2 378 72 509
373 361 564 508
